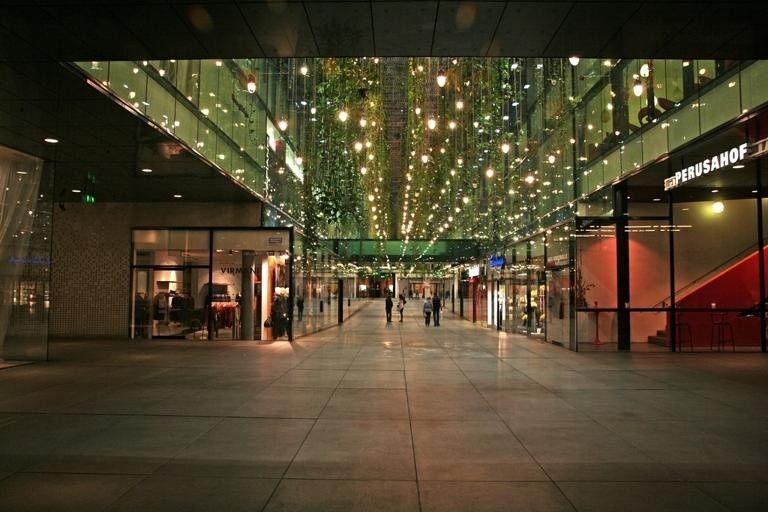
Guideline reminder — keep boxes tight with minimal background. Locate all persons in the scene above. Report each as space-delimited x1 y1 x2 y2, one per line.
423 297 433 327
408 289 413 300
296 296 304 321
385 296 393 322
397 294 405 322
431 292 442 327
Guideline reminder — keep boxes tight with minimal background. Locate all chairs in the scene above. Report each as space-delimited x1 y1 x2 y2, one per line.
706 310 736 352
673 304 695 352
